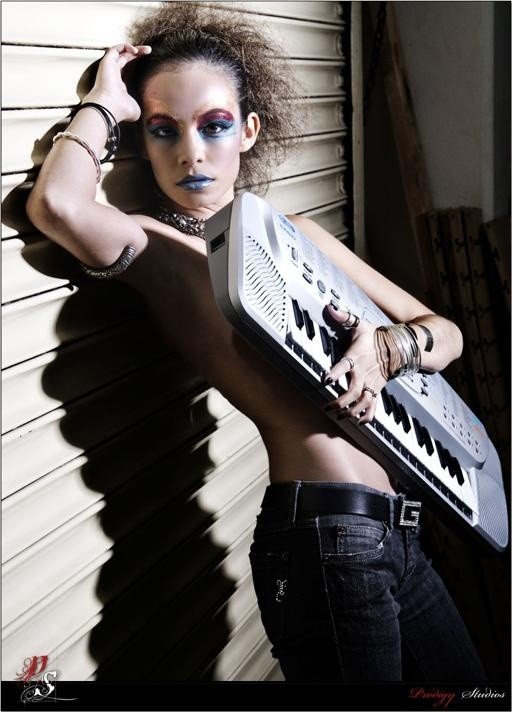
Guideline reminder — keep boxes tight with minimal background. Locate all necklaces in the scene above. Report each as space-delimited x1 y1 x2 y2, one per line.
152 200 207 243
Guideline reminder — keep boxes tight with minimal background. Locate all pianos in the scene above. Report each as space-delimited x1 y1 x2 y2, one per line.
205 192 508 549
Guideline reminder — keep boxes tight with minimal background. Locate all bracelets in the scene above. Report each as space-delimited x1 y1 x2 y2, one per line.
69 102 120 164
376 323 422 381
51 132 101 184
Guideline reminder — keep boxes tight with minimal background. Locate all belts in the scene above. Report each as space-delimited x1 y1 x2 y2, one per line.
260 485 422 529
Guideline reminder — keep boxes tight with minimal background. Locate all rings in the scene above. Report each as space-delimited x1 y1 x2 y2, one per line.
341 312 353 327
350 314 360 328
340 357 354 372
362 387 375 398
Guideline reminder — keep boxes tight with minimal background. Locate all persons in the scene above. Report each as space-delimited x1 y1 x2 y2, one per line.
27 1 491 684
